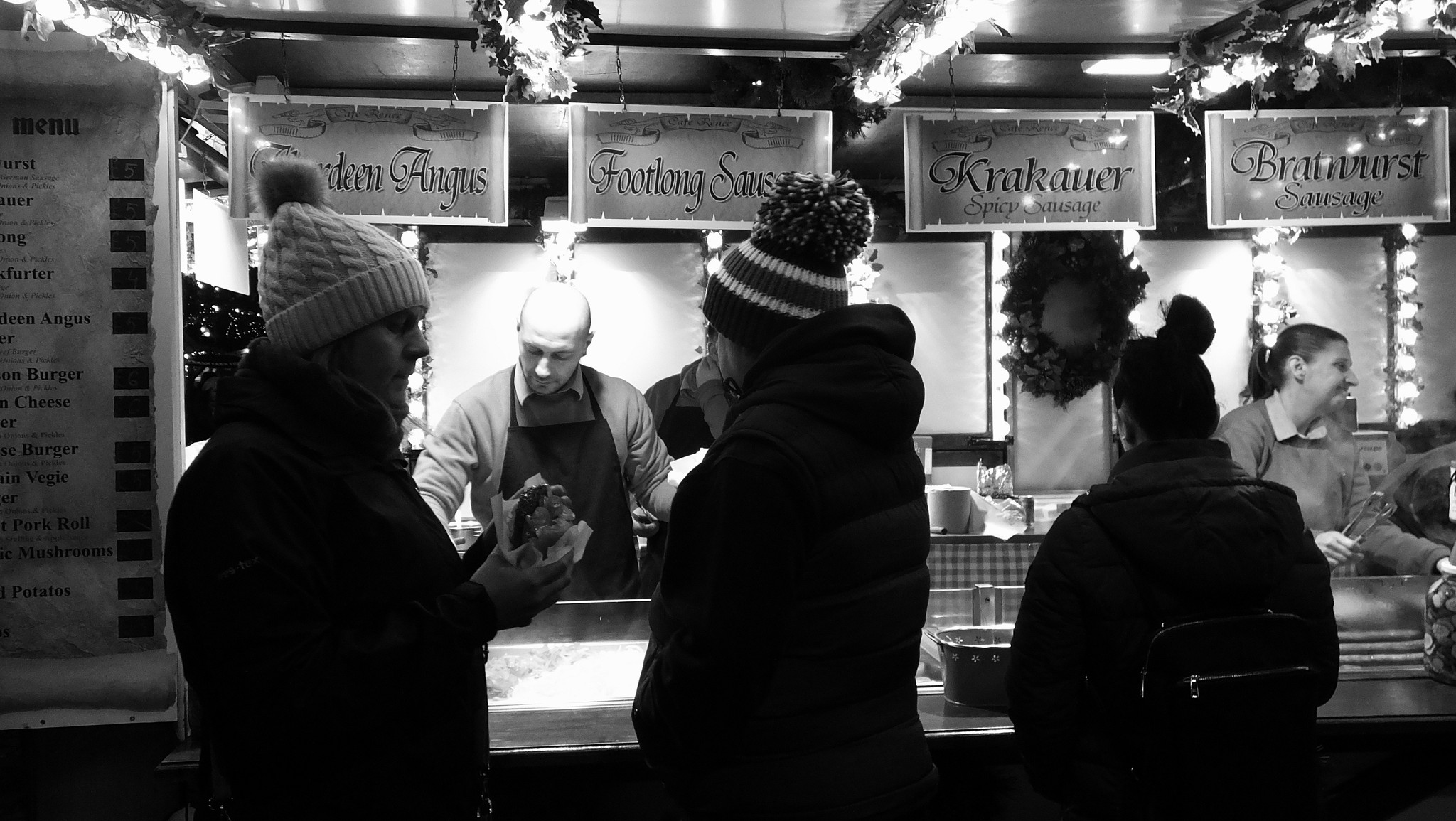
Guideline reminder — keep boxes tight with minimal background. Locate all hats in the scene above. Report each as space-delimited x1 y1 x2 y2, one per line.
701 169 877 351
248 155 432 354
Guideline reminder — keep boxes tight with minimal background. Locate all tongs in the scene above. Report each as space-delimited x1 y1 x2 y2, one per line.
1342 492 1398 545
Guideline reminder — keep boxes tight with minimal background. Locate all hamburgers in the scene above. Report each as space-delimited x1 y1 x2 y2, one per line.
507 484 574 560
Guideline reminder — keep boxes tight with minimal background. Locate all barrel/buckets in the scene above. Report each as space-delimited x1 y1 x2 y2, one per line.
925 628 1014 707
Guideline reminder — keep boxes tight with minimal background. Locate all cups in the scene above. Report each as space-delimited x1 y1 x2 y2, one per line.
928 487 971 533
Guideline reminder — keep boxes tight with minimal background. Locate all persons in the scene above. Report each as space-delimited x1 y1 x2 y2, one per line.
411 283 679 601
1006 295 1341 820
633 171 948 821
1209 324 1451 578
165 157 575 821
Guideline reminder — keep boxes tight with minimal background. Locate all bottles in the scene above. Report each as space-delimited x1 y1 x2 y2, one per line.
1423 556 1456 685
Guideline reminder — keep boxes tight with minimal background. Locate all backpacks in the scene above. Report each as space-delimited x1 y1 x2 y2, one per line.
1108 535 1317 821
